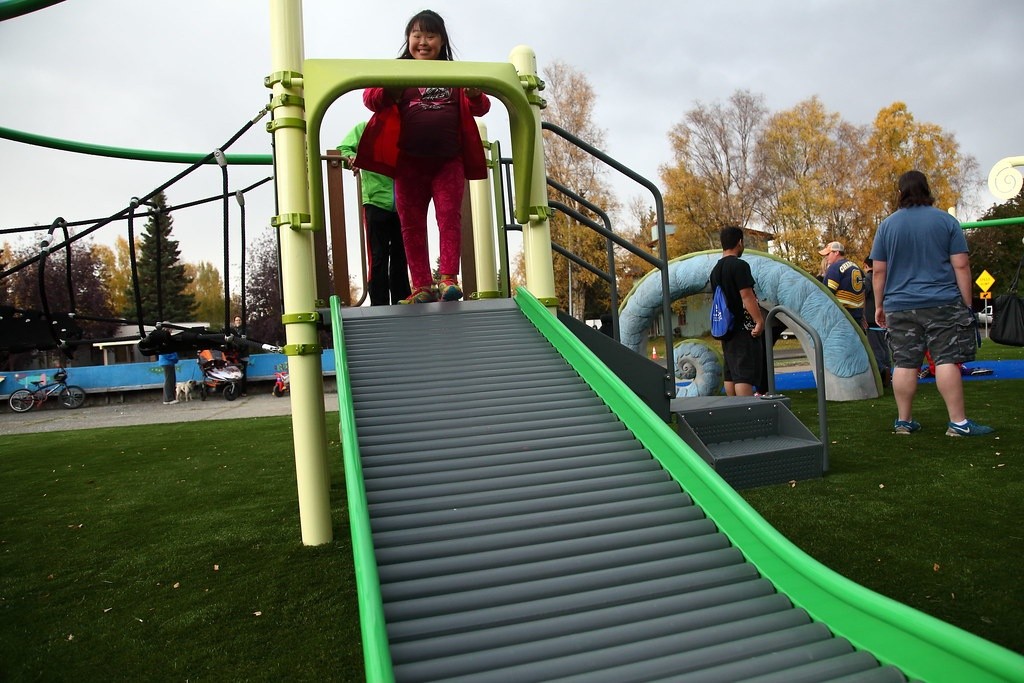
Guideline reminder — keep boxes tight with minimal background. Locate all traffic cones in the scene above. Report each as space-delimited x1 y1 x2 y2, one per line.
651 346 658 359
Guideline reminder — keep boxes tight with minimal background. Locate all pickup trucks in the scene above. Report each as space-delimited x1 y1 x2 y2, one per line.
974 305 993 324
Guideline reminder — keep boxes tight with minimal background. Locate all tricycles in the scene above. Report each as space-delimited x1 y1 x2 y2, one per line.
273 371 289 396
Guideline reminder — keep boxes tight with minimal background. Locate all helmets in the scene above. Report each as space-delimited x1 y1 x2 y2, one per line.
54 373 68 381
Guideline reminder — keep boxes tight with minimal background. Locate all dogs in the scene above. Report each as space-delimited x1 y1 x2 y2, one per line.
175 380 196 401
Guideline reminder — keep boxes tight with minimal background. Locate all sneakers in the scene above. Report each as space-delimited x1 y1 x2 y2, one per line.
894 419 922 434
436 279 464 301
945 420 995 437
397 286 438 304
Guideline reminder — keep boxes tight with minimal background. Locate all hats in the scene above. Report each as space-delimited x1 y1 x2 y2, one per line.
818 241 845 255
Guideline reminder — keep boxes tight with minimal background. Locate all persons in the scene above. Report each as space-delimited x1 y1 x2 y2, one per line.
815 241 891 387
710 226 764 397
159 322 179 404
869 170 995 436
335 122 412 306
234 315 249 396
352 9 491 305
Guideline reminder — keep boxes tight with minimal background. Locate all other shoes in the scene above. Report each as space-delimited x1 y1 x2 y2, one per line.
163 400 180 405
880 367 891 388
241 392 246 397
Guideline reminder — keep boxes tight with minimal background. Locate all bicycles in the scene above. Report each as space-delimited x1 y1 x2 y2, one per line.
8 371 86 413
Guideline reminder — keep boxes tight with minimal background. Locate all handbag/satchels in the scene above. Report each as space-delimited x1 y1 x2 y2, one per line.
990 260 1024 349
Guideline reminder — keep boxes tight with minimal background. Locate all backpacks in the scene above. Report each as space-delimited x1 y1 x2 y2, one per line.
709 265 735 338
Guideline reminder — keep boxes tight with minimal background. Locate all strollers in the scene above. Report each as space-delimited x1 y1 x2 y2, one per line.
192 345 245 401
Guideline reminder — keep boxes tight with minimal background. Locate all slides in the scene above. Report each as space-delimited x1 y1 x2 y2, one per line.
329 283 1023 683
